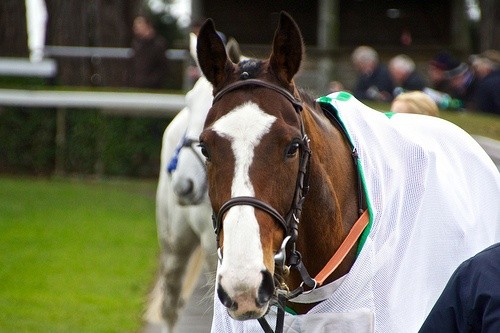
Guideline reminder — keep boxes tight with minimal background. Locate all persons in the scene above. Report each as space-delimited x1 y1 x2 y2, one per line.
123 15 170 177
332 46 500 118
418 241 500 333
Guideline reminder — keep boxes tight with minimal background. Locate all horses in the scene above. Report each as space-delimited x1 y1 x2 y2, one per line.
139 31 219 333
196 10 499 333
